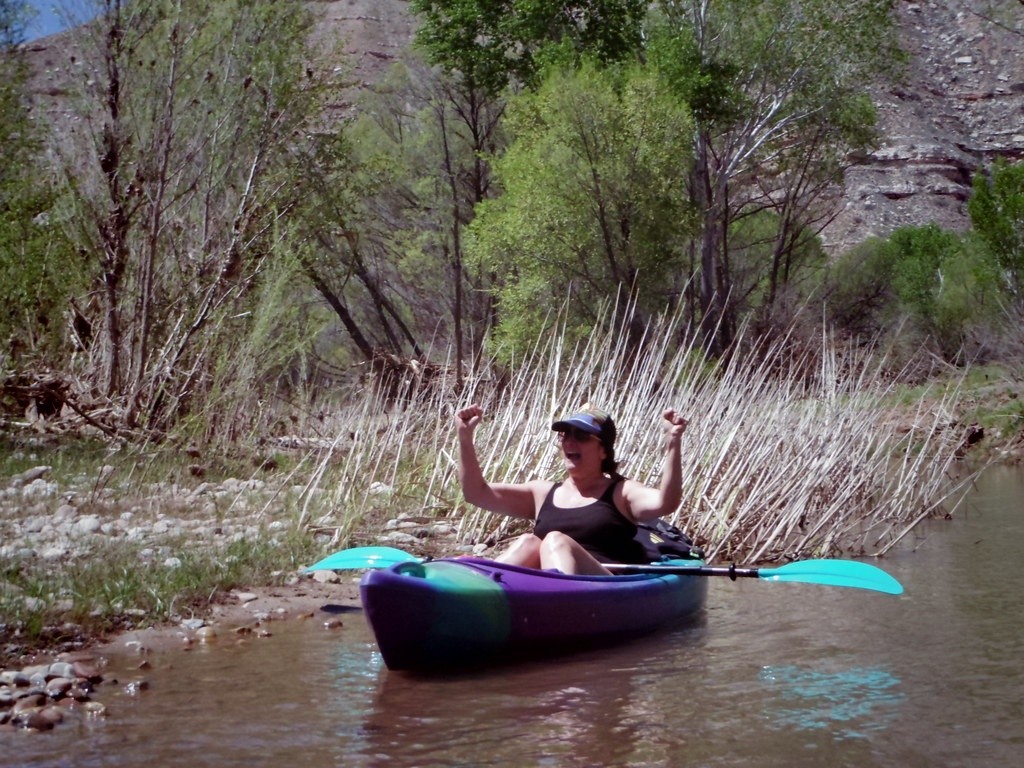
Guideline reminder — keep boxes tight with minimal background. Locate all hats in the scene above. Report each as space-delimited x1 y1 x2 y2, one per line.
552 403 617 446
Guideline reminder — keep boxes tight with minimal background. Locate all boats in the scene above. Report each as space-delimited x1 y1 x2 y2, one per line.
359 518 710 677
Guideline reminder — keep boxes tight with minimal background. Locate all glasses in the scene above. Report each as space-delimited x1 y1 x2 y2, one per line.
555 428 602 444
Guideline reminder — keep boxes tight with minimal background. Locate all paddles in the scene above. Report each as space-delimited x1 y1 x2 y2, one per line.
305 545 902 595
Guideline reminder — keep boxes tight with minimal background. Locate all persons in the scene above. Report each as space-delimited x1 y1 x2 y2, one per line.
456 402 688 577
